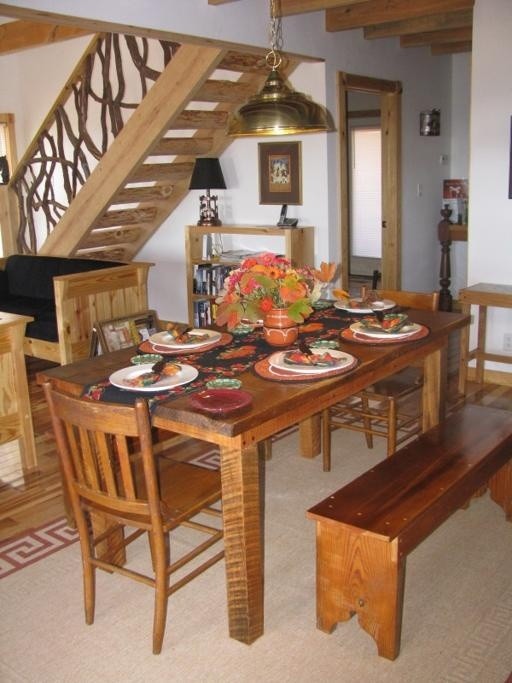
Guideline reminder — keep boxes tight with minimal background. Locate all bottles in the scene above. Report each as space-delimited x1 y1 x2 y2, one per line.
359 283 367 297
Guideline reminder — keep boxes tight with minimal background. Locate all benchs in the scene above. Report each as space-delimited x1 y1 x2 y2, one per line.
303 399 511 661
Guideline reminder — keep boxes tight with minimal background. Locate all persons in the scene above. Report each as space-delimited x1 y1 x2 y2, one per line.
271 158 289 183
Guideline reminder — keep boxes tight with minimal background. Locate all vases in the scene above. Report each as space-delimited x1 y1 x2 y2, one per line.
261 303 302 346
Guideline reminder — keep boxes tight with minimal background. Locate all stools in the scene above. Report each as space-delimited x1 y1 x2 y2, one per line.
454 280 511 399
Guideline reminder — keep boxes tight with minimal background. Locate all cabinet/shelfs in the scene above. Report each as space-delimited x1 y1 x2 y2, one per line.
184 221 316 335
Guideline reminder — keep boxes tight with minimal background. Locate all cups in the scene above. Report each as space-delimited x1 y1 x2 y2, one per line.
365 288 378 305
207 233 224 260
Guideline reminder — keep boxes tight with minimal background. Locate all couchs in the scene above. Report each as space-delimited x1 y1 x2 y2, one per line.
0 252 157 368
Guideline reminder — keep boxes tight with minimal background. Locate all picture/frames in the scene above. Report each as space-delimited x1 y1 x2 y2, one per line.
257 140 304 206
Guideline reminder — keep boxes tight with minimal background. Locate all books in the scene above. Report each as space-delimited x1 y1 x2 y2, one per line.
192 261 240 327
218 247 286 263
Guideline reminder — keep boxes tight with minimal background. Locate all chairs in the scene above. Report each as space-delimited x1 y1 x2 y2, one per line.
314 284 442 474
40 376 227 654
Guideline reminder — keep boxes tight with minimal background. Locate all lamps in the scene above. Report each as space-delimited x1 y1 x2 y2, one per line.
188 155 228 227
225 0 338 138
419 108 441 137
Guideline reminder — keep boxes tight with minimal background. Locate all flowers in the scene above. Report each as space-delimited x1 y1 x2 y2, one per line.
214 246 327 328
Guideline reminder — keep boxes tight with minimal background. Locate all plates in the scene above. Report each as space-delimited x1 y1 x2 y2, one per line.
239 313 265 328
190 389 255 414
266 347 356 373
349 319 424 338
148 327 222 349
205 378 242 390
129 353 165 365
108 361 199 392
332 295 398 314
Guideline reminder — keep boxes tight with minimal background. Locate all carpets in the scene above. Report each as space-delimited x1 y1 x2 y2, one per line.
40 309 510 478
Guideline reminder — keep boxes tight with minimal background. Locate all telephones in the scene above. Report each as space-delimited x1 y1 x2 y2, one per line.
277 204 299 226
